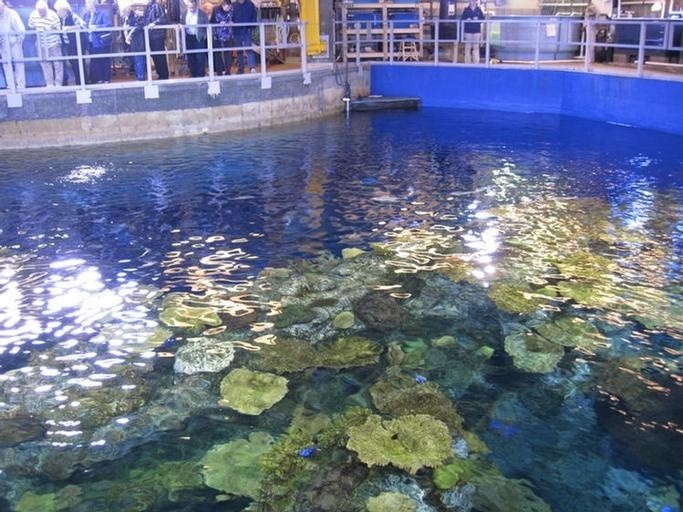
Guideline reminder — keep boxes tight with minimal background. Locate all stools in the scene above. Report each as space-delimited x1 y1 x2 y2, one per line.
396 35 418 62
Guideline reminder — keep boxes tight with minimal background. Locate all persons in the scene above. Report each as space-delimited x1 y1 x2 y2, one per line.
28 0 258 88
0 0 27 90
462 1 485 65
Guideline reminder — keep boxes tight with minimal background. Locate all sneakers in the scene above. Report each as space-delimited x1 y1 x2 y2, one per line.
237 69 244 74
251 68 256 74
